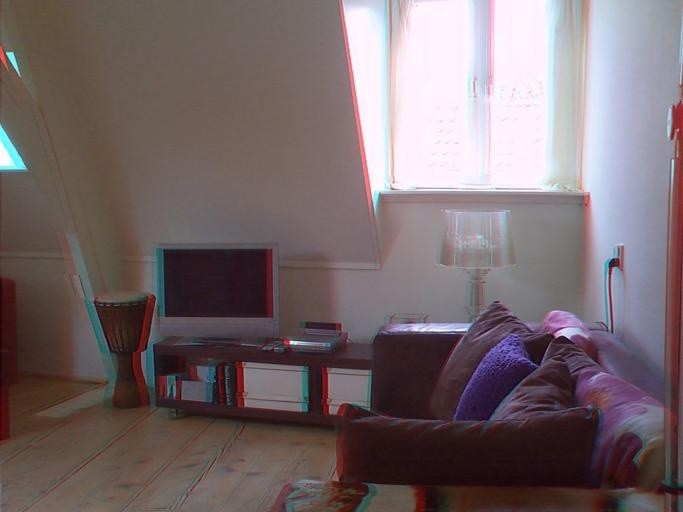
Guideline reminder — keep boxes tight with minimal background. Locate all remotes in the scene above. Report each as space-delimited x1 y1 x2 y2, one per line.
275 345 290 353
261 340 283 352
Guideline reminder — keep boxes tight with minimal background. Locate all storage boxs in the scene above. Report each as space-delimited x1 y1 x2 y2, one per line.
237 393 308 413
322 367 371 401
236 361 309 396
320 399 372 415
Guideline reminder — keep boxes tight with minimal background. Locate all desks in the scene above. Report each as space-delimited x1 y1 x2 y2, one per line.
153 336 374 426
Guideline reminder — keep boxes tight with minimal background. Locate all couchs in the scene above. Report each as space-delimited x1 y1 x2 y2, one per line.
270 322 666 512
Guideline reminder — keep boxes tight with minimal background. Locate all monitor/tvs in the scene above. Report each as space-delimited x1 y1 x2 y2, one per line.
155 243 279 347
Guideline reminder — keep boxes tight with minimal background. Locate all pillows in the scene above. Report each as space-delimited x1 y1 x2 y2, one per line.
454 333 539 421
429 299 555 420
575 369 677 488
541 336 600 373
541 310 598 358
336 403 599 485
489 356 574 420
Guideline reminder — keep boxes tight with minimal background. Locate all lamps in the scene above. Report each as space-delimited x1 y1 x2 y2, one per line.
437 208 516 323
